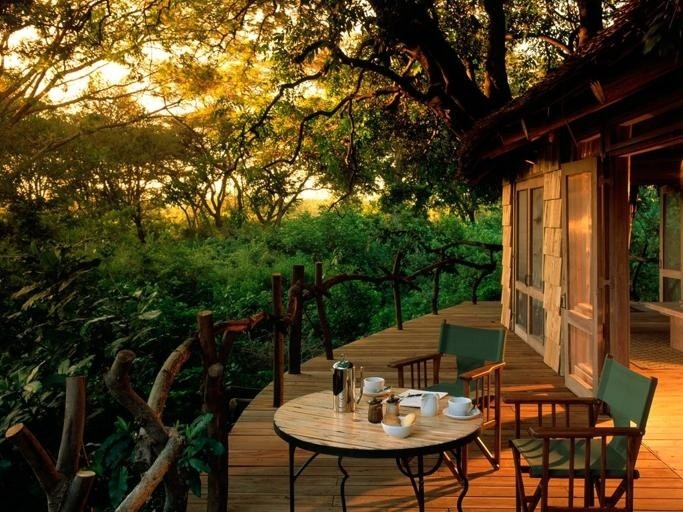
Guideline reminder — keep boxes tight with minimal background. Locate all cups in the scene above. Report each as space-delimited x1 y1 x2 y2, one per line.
448 397 476 416
365 377 385 394
419 392 440 418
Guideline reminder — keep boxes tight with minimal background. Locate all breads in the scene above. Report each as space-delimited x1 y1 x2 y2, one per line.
383 412 416 426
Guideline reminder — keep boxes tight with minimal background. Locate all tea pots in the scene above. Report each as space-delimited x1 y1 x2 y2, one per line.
330 353 364 414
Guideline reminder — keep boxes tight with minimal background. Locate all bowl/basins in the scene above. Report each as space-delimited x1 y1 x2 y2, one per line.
381 416 416 440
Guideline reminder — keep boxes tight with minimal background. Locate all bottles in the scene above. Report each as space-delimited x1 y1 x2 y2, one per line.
368 395 401 424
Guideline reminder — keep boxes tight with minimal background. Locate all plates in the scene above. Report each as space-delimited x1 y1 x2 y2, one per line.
361 387 391 399
442 407 481 420
396 389 448 408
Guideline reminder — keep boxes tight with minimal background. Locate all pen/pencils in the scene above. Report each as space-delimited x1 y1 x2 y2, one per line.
407 394 422 397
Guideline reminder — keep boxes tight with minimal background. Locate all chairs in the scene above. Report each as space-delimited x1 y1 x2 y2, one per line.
388 318 508 489
503 353 657 512
